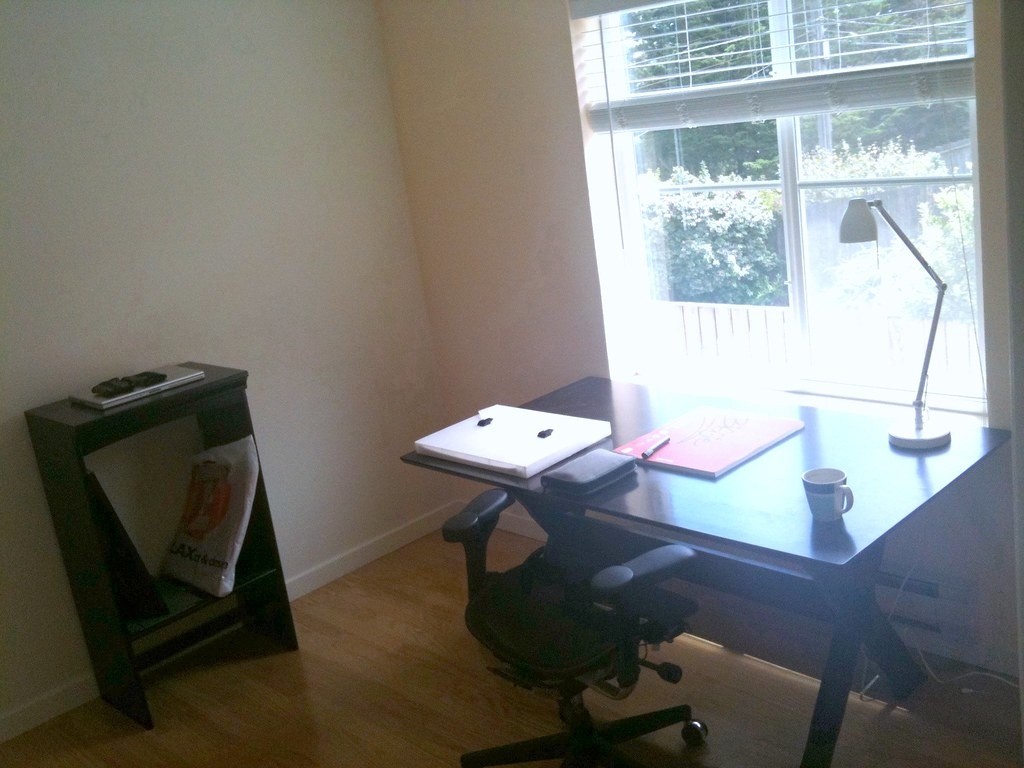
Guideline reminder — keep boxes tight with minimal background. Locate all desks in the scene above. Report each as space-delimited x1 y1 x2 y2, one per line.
399 375 1017 768
24 361 302 734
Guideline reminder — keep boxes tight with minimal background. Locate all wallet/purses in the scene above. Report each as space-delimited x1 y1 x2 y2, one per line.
541 448 637 497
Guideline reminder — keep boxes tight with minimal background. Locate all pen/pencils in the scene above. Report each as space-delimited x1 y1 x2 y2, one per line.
642 436 671 460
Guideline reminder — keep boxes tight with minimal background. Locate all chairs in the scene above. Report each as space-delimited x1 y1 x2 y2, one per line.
440 481 710 767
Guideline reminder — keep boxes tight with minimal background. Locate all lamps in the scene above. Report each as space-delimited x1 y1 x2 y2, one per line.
838 197 958 452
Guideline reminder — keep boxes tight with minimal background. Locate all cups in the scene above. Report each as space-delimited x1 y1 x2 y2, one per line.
802 467 853 521
